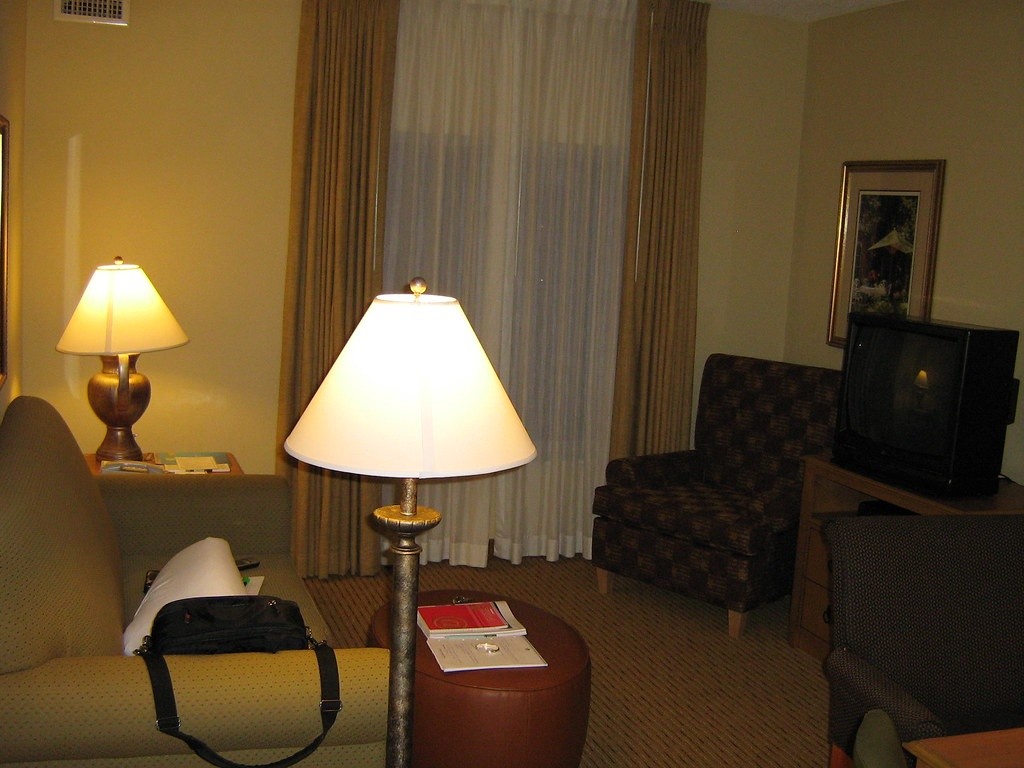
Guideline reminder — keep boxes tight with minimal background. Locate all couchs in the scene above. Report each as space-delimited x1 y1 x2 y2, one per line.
1 396 390 768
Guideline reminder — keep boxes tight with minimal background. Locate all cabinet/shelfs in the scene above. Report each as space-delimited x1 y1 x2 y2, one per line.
789 451 1024 661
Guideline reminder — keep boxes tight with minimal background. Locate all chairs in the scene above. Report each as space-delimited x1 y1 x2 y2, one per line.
591 353 843 638
823 515 1024 768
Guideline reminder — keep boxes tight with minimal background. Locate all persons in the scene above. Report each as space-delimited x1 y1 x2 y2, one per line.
853 270 907 313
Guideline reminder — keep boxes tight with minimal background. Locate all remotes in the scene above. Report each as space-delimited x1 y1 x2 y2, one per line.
235 557 260 570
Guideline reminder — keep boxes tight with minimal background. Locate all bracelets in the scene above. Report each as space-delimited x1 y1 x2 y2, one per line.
475 642 499 654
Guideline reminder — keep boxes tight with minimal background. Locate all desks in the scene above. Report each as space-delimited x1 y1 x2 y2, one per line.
902 727 1024 768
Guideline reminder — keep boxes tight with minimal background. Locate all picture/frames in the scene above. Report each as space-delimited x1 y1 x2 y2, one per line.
0 114 10 391
825 159 945 350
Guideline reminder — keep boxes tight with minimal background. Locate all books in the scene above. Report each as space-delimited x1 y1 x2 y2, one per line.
419 599 546 674
101 450 231 474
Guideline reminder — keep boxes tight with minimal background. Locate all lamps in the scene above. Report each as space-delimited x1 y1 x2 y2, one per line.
55 257 191 464
283 278 538 768
914 371 928 411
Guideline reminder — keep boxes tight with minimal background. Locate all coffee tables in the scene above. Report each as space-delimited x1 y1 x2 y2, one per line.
369 589 593 767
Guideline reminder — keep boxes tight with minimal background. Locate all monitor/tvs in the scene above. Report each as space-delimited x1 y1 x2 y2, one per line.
831 311 1019 500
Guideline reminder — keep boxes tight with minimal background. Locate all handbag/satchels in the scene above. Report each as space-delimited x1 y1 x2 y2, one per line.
141 595 343 767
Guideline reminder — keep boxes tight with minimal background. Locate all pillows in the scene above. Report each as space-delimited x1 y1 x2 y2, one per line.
123 536 248 657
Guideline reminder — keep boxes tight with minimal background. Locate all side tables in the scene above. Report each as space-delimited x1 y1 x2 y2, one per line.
82 452 244 476
